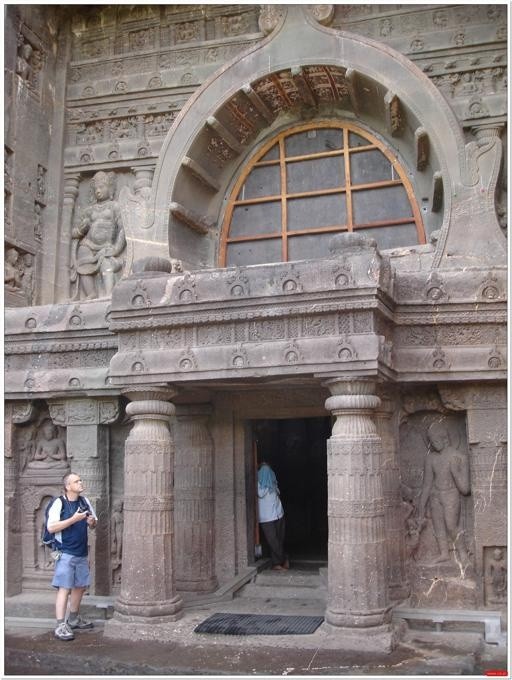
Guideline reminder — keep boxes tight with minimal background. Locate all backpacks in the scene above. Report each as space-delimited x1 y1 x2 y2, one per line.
43 496 58 551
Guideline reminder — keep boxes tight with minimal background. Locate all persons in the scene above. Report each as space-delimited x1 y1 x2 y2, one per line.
108 498 126 561
44 471 98 643
414 417 471 567
69 170 127 299
26 424 69 471
485 545 508 603
255 458 292 571
4 248 24 292
19 253 34 289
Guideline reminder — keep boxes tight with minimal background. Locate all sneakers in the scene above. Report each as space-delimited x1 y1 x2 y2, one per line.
67 617 94 629
53 622 75 641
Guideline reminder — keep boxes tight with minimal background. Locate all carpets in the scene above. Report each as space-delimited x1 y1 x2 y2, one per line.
193 609 329 638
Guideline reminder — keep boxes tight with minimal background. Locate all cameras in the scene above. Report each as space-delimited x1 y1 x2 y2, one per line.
79 501 89 513
